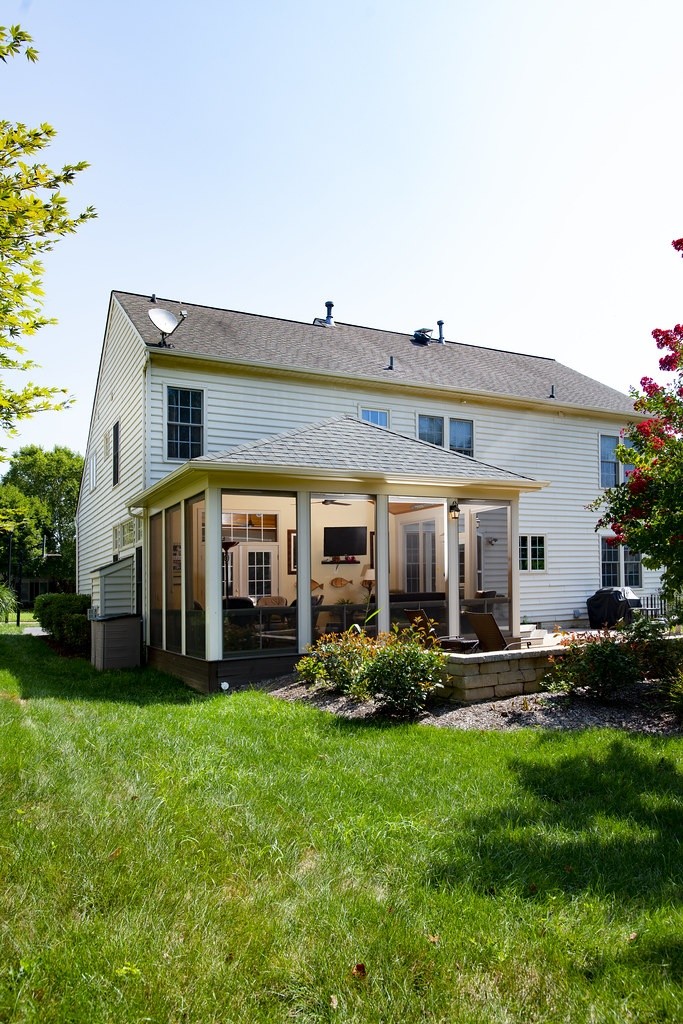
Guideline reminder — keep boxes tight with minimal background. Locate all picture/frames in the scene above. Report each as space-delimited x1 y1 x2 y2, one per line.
287 529 298 575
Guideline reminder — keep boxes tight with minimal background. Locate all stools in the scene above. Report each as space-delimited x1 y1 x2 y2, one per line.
654 618 669 631
650 620 666 633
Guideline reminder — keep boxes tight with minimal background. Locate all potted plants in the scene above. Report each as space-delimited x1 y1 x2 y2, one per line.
329 598 357 630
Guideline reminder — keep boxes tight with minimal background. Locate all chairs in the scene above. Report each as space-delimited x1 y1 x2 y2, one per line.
222 596 258 651
322 622 344 643
194 600 204 610
460 590 497 617
404 609 479 653
285 594 323 630
465 611 531 654
255 596 289 633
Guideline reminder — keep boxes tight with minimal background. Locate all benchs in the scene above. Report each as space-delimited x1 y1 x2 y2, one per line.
364 592 445 626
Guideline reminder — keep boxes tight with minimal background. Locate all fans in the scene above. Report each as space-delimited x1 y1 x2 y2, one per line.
290 500 351 505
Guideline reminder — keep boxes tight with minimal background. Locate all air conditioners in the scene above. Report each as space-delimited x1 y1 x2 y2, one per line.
87 609 97 620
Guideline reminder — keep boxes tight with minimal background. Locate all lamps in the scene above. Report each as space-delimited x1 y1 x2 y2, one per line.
248 514 254 527
449 500 460 520
363 569 375 603
221 535 240 609
476 513 480 528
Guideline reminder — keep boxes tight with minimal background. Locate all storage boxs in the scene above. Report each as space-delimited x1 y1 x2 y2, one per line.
520 622 542 629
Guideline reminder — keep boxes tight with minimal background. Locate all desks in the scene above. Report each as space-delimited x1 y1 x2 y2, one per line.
630 607 661 621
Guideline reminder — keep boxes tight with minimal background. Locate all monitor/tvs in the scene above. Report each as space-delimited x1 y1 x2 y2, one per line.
323 526 367 557
